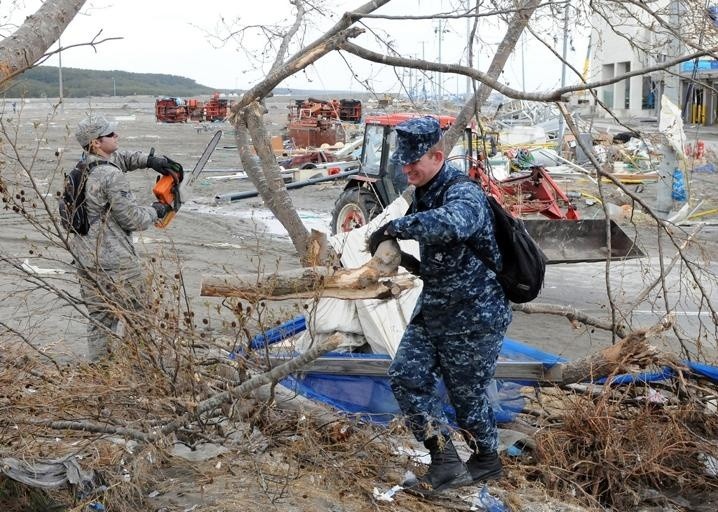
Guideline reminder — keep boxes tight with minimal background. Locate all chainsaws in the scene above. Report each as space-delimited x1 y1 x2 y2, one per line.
152 130 222 228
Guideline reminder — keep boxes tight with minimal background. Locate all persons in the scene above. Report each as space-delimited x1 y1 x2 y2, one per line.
372 116 512 492
60 116 186 396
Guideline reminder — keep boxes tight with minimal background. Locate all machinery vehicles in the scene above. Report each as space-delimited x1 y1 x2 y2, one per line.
327 109 646 265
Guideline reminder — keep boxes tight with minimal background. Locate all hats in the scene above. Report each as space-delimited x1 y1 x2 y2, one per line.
389 115 443 165
74 112 119 148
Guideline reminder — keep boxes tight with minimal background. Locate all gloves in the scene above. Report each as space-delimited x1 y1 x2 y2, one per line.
146 147 184 186
368 220 420 276
151 201 169 219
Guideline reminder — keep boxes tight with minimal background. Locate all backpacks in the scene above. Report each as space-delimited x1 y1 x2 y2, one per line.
58 151 120 236
434 177 546 304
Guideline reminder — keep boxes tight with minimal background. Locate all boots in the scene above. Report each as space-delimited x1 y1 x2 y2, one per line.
403 431 474 497
465 450 503 484
